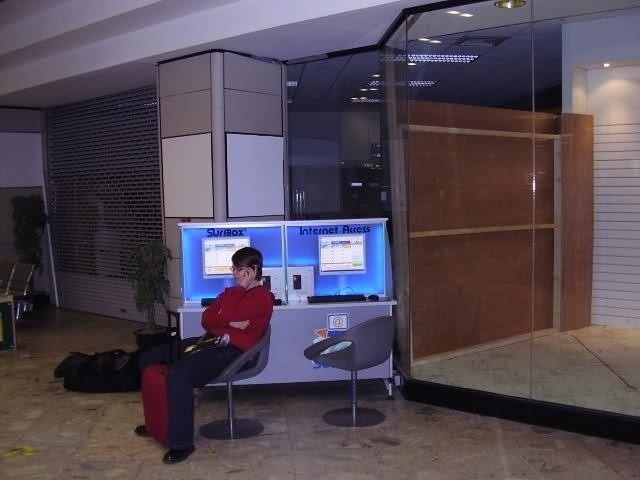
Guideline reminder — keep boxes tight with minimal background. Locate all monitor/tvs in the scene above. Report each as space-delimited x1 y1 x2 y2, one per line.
318 234 366 275
202 236 251 280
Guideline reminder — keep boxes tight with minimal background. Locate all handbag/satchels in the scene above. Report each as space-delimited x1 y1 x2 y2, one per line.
54 349 139 393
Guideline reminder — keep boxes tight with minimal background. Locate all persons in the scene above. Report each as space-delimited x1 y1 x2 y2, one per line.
131 245 275 465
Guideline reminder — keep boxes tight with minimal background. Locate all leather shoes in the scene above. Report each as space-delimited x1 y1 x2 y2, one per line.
133 425 148 437
161 444 196 465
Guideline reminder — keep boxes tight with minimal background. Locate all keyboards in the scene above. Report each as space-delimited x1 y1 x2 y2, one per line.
201 298 217 306
307 294 366 304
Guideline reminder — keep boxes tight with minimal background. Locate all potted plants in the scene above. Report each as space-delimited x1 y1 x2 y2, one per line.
9 194 50 311
125 238 173 350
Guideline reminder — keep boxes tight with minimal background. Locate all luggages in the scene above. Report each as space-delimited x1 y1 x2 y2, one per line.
140 309 182 446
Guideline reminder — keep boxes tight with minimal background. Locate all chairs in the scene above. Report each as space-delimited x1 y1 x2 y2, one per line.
1 262 37 322
200 324 270 440
303 316 395 427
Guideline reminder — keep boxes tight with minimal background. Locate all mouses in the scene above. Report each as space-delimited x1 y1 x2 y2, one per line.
276 298 282 305
367 294 379 301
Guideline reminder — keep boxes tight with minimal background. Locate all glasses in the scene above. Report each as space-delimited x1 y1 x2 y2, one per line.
230 266 249 272
